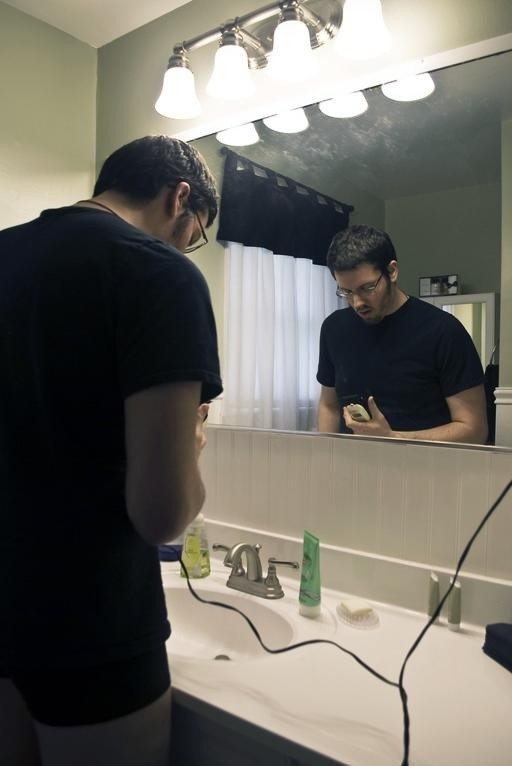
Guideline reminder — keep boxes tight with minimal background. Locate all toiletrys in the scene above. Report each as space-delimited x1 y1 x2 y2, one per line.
427 572 440 621
446 578 462 631
300 532 322 617
181 513 210 578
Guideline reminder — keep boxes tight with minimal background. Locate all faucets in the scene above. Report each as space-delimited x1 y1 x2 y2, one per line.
223 543 262 581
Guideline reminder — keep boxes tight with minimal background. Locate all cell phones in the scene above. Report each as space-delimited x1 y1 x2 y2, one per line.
342 396 372 421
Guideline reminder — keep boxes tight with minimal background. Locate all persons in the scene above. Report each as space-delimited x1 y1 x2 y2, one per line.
2 137 221 759
316 225 488 443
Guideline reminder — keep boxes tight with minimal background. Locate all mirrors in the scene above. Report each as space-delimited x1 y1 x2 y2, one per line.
156 28 511 452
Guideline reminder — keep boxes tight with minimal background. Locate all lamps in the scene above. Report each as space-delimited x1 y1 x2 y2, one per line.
214 71 437 149
155 0 345 125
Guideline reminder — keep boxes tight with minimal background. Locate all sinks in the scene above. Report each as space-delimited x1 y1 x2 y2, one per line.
162 586 293 663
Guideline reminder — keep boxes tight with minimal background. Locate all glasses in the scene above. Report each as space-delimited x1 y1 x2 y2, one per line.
336 273 385 298
166 181 208 254
